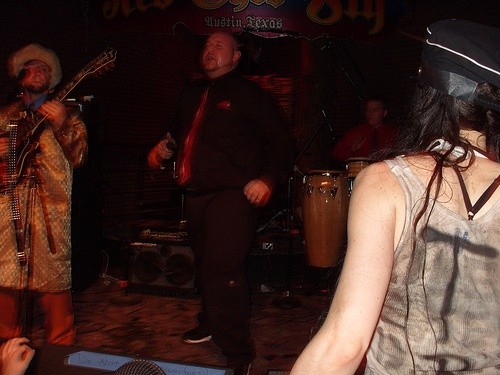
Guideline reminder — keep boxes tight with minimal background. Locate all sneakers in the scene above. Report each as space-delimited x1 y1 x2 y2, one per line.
182 327 212 344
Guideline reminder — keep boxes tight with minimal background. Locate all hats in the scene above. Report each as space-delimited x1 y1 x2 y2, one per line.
418 18 500 112
7 43 63 95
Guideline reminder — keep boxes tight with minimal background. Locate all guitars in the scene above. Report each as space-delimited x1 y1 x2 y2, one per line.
0 47 118 196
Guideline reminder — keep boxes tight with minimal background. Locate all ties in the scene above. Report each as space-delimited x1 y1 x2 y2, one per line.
175 83 215 186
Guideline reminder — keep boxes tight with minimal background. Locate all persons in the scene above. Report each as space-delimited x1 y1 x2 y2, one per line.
331 93 406 164
0 44 89 348
146 30 296 375
0 336 36 375
289 18 499 375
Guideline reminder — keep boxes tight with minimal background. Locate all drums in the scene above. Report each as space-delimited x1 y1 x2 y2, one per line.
300 168 351 269
342 157 376 195
